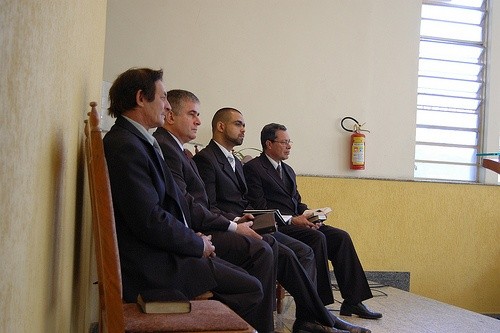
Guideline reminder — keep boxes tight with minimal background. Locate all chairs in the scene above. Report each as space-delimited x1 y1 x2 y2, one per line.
82 101 285 333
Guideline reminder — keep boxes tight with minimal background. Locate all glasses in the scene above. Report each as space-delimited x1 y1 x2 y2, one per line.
271 139 292 146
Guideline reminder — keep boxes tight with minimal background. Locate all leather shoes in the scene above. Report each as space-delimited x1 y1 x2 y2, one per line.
340 299 383 319
328 312 371 333
292 318 351 333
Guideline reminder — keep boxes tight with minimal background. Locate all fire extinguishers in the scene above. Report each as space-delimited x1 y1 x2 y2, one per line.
341 117 370 170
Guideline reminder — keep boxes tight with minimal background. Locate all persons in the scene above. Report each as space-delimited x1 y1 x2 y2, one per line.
193 108 370 333
244 122 383 320
101 68 264 319
152 90 278 333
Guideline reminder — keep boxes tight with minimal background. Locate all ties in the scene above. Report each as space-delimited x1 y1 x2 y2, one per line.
183 149 189 159
277 165 282 181
154 138 164 159
228 154 235 173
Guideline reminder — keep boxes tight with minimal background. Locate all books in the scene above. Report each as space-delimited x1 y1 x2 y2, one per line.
249 212 277 234
245 208 292 225
308 215 326 224
